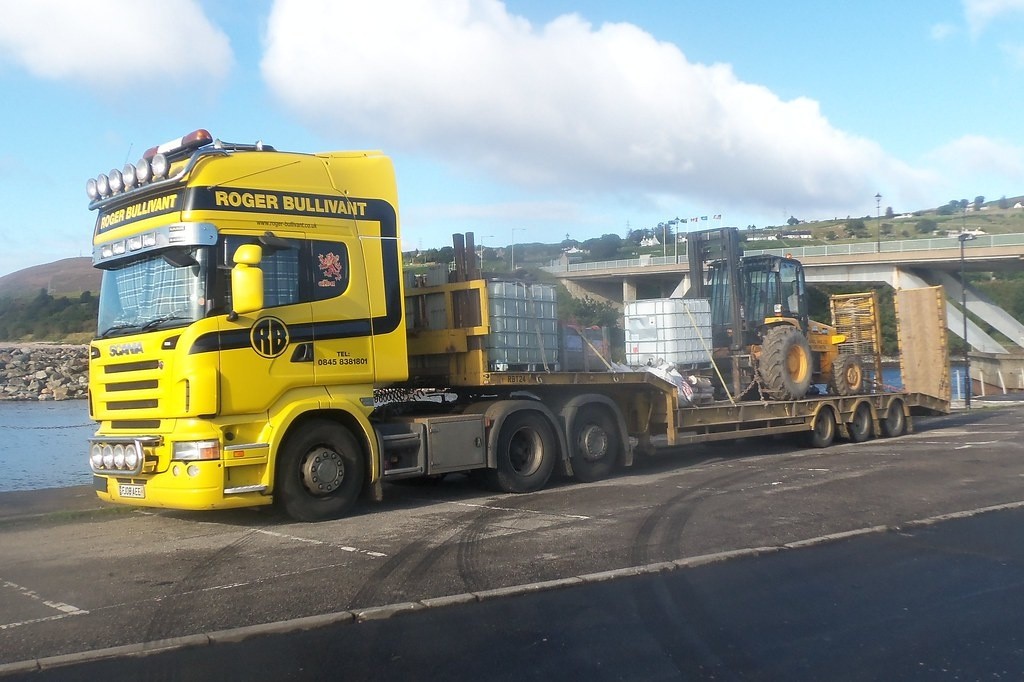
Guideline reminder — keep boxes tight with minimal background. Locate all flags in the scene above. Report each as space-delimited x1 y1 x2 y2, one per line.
690 217 697 222
713 215 721 219
701 216 707 220
668 220 675 224
680 219 687 223
658 222 663 226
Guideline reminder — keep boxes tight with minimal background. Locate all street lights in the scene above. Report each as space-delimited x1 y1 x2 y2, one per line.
511 228 526 271
958 233 977 408
566 233 569 272
675 216 680 264
751 224 756 245
481 236 494 268
875 192 882 253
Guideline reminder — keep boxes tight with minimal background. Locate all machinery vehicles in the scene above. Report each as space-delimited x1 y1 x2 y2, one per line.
687 227 863 401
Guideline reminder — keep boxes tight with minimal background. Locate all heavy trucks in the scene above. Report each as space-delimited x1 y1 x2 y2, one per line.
86 128 951 523
